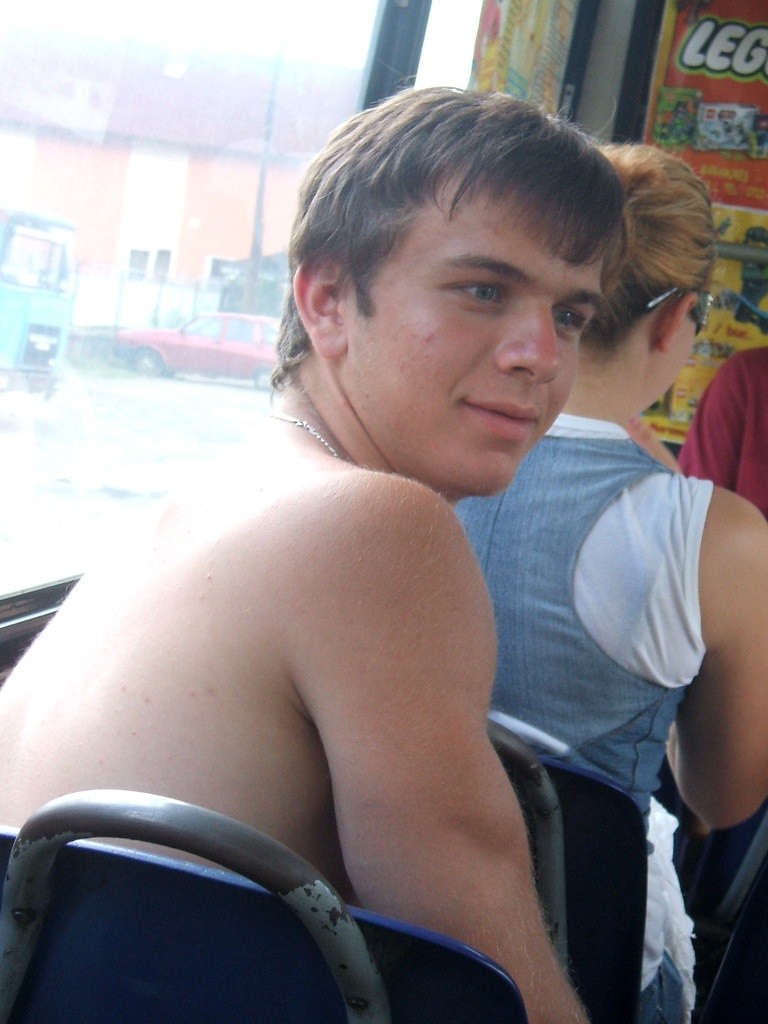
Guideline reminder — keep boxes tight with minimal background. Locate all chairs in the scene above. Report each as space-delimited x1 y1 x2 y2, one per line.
0 789 530 1024
483 718 648 1023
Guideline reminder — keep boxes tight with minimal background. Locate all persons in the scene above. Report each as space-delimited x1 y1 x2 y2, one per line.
453 143 766 1019
677 342 766 527
0 85 598 1024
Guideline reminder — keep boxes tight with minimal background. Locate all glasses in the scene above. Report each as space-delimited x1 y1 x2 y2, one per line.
644 286 712 334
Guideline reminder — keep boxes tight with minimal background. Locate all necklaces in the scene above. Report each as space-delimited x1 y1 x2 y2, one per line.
264 407 345 463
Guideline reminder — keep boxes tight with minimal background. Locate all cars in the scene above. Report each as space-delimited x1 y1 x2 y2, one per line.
115 310 284 385
